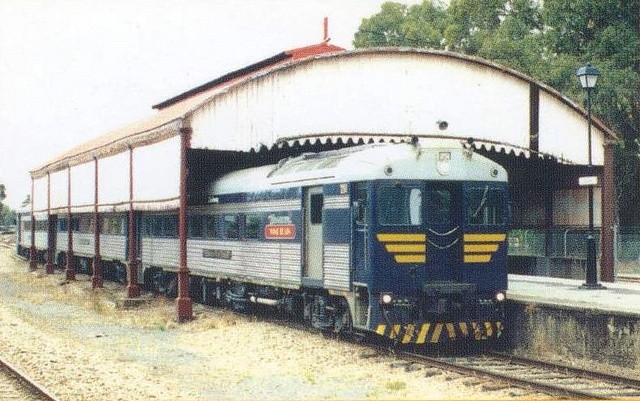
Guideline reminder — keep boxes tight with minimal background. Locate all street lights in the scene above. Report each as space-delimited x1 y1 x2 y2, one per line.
576 62 607 291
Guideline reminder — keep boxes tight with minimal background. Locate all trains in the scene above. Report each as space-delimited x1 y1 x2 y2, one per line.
16 138 509 355
